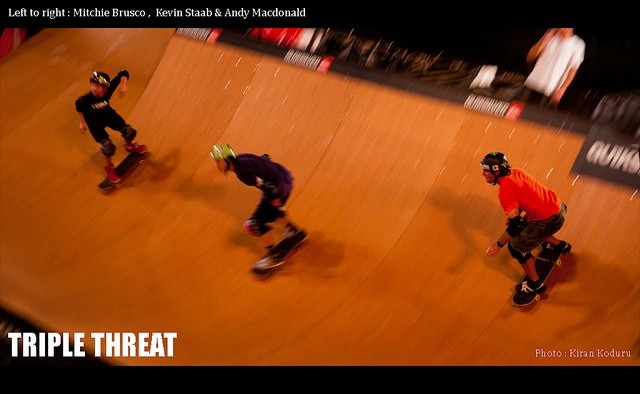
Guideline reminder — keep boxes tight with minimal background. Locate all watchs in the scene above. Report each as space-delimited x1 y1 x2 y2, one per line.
496 240 504 248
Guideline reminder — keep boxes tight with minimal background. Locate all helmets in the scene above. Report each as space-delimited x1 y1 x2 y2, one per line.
209 143 236 158
89 71 111 86
480 152 510 171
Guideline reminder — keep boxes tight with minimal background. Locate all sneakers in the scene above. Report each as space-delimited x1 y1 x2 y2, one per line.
541 241 571 253
253 253 284 269
284 225 301 238
124 142 147 154
105 162 121 184
515 281 546 296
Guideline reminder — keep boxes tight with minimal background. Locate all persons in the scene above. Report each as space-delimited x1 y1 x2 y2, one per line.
75 70 147 184
512 28 585 106
467 151 568 305
209 144 303 272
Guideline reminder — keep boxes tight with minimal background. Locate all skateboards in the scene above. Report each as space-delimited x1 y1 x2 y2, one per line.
512 241 563 308
97 150 146 190
253 229 309 274
